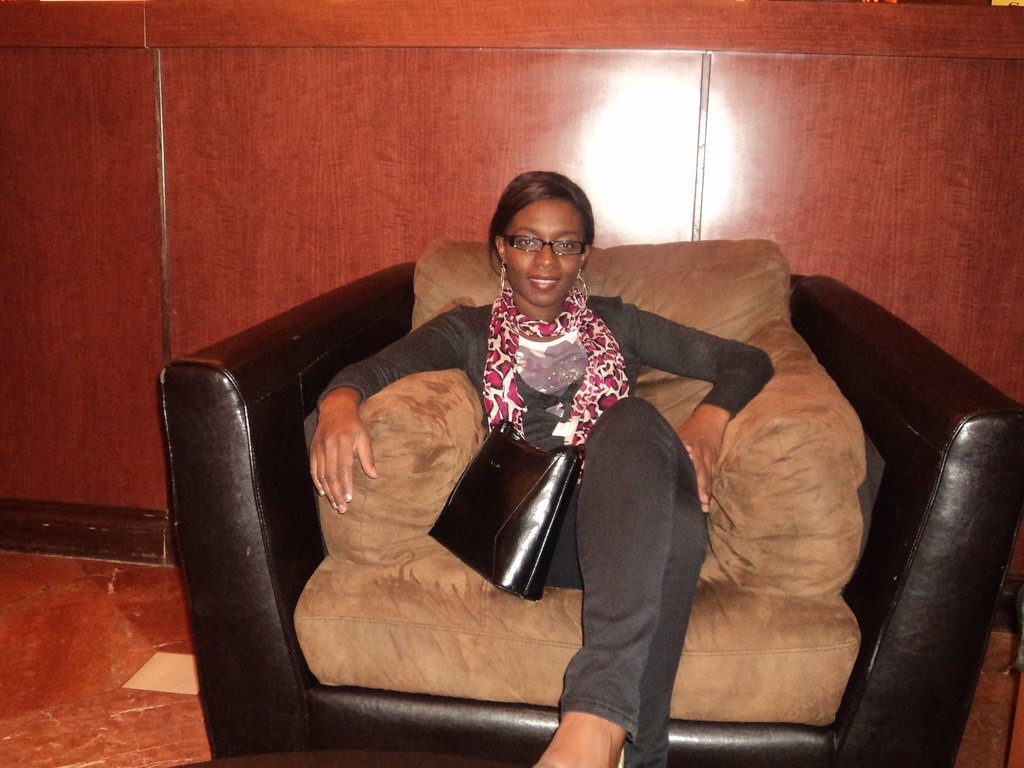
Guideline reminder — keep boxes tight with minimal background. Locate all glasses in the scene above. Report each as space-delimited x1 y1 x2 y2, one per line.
499 233 593 255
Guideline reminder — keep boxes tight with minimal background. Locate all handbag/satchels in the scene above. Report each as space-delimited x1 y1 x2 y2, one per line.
427 419 587 601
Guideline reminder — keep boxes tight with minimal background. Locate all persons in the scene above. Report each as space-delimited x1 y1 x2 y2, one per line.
309 171 773 768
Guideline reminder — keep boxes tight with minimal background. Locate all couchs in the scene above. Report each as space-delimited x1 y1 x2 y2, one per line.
158 238 1024 768
